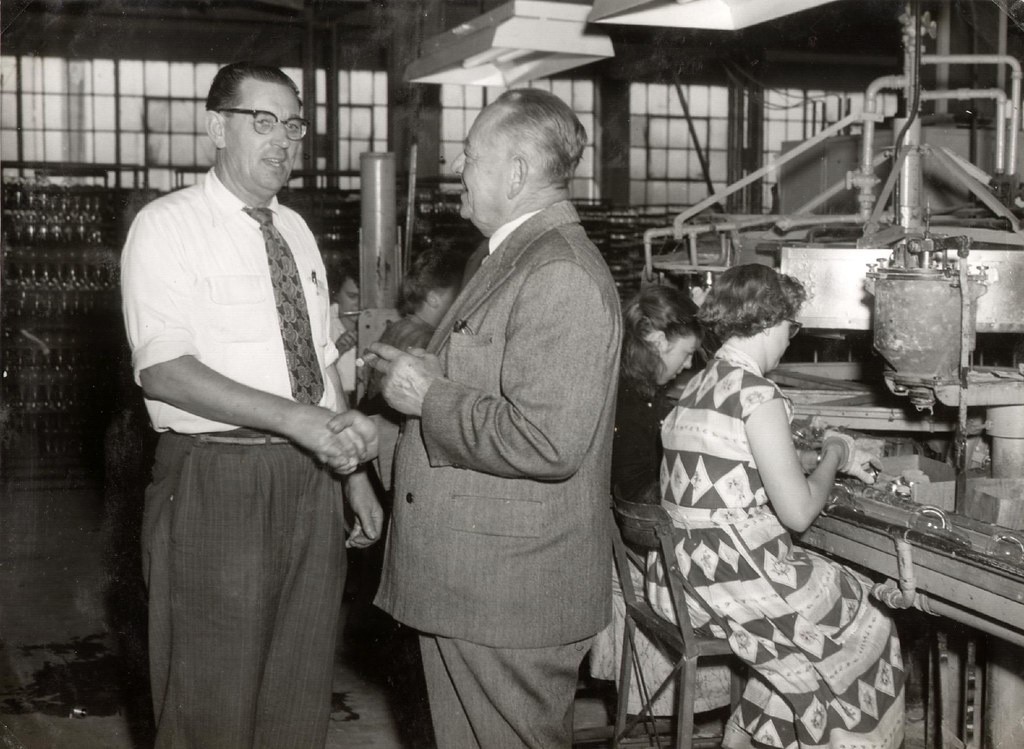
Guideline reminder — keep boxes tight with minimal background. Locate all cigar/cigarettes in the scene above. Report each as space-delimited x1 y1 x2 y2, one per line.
355 353 377 367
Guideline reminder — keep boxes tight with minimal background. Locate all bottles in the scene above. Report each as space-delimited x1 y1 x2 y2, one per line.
0 187 121 457
284 186 462 302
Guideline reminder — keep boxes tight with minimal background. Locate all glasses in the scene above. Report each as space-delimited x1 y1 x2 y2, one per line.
453 318 473 335
218 108 311 141
783 318 803 338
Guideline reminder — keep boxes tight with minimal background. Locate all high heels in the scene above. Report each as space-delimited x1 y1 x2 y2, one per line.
720 721 770 749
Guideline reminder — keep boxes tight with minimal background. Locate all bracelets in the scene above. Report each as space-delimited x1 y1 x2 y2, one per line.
820 436 849 470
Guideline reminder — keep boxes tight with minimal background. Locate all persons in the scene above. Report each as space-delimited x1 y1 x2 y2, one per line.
358 229 491 436
330 245 360 414
316 88 625 749
645 265 905 749
119 59 383 749
611 284 704 557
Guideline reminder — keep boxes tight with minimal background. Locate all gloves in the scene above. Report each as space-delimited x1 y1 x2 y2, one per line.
820 430 883 484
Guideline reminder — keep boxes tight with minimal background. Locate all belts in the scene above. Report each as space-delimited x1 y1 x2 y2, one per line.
189 434 293 446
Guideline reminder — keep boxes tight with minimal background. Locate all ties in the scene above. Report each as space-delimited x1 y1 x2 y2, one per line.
243 207 325 407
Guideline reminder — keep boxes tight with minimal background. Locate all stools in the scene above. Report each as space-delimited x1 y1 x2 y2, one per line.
606 492 749 748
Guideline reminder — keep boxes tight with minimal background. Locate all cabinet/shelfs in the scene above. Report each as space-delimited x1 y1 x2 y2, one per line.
276 186 363 295
0 163 128 482
573 197 688 301
399 175 481 281
